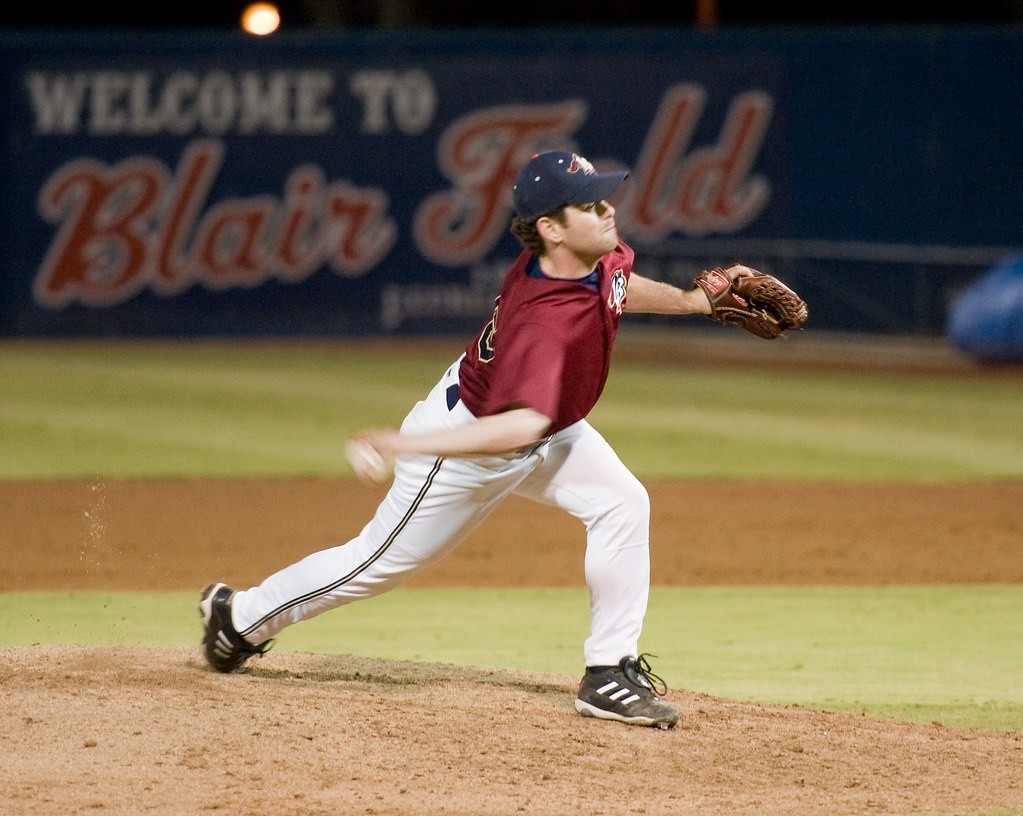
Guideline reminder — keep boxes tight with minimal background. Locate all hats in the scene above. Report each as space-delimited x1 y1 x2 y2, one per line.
512 151 630 221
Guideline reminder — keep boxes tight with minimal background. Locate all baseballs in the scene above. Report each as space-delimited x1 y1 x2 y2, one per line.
347 440 386 481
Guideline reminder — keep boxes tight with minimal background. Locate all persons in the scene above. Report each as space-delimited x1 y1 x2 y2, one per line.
199 150 809 724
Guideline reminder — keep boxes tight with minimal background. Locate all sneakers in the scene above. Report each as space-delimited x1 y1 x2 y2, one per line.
574 653 679 728
199 582 277 674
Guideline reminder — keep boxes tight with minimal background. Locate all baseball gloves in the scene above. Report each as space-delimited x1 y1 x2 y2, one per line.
691 262 809 340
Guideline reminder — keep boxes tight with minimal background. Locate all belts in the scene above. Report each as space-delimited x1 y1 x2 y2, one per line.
446 383 461 412
